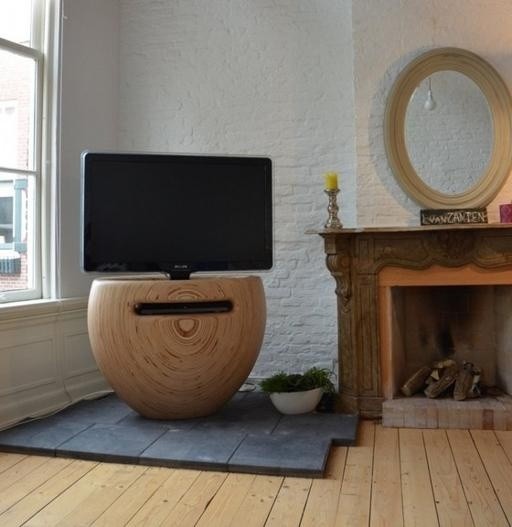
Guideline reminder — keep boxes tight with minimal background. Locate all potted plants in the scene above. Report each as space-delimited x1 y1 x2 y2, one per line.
258 367 336 415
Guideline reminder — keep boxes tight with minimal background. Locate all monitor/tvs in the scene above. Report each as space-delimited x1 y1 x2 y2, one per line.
80 148 275 280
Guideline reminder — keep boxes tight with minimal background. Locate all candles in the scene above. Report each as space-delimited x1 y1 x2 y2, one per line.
325 172 337 190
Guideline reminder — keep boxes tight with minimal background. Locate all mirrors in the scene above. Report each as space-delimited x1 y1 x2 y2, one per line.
383 48 512 210
